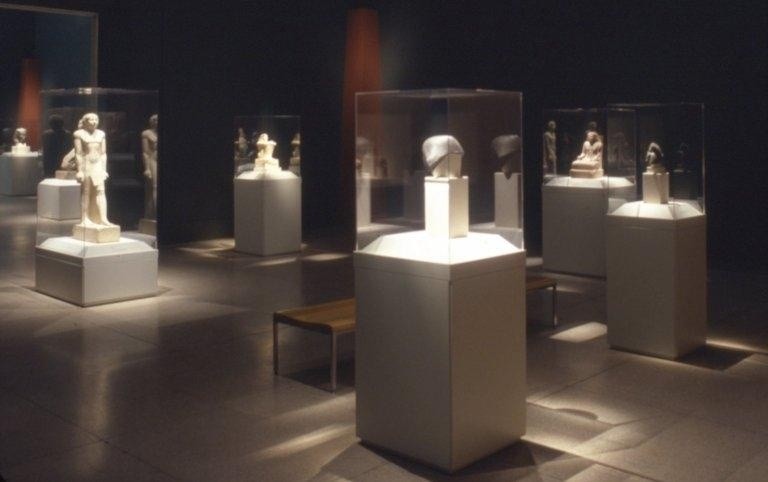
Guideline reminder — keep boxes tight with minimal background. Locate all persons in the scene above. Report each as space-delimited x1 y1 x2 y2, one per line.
142 113 157 217
543 120 557 173
12 128 26 145
42 114 75 181
580 131 603 160
72 112 108 226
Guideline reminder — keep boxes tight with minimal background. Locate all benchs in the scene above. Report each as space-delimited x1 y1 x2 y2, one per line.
273 276 558 390
